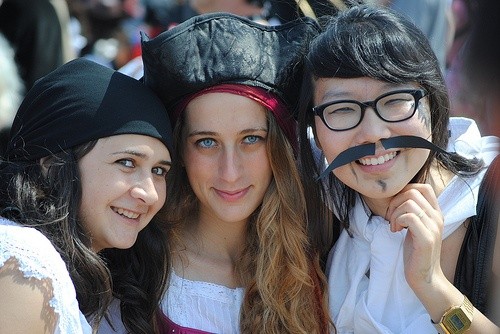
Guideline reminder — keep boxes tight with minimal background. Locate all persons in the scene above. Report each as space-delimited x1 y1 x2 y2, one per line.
0 57 173 334
293 3 500 334
98 13 346 334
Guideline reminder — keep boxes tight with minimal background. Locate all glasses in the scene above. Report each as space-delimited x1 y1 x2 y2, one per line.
310 90 430 131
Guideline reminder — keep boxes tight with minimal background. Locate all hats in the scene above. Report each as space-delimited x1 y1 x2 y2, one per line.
140 12 324 153
10 58 172 161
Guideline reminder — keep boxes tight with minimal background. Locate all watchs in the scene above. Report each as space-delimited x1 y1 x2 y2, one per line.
430 296 474 334
